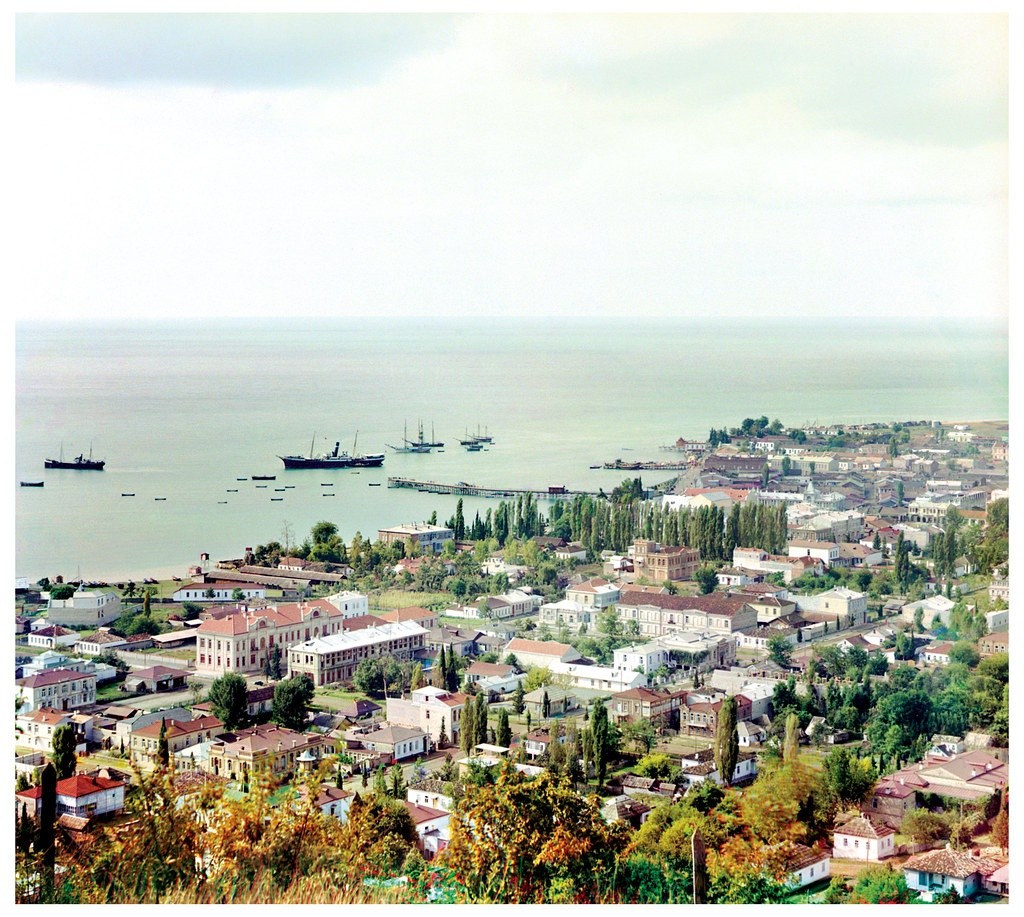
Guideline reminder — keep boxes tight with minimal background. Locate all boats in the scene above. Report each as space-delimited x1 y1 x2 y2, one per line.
44 440 105 471
274 426 385 468
384 417 497 453
